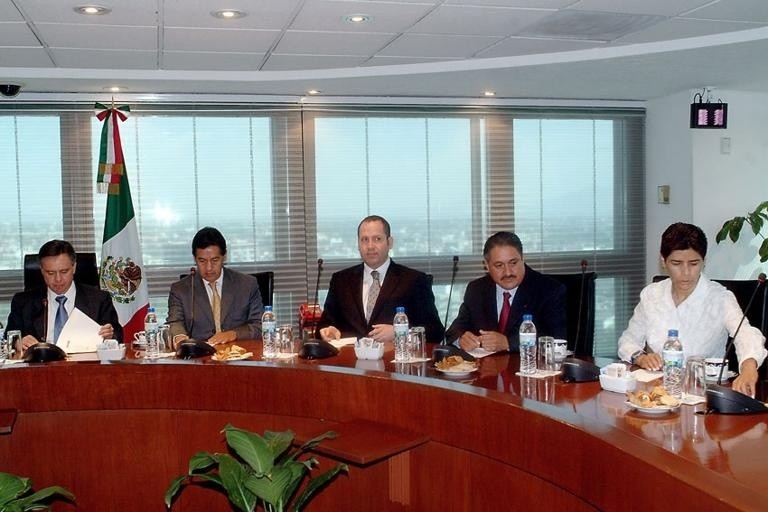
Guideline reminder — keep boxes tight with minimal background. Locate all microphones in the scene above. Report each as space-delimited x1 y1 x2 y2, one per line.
706 273 767 415
176 267 217 358
298 259 340 357
433 255 476 366
21 298 67 363
560 258 601 382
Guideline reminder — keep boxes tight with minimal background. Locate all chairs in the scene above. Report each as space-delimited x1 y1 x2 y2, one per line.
179 270 274 311
23 252 100 294
538 270 598 359
652 275 768 381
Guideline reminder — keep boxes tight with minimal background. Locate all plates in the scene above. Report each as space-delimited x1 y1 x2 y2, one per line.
627 399 684 416
438 364 478 376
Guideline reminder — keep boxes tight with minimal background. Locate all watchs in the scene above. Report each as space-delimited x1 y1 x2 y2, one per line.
630 350 646 364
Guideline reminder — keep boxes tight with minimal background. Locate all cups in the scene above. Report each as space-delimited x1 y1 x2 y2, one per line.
0 326 22 367
539 375 555 406
274 325 295 355
687 401 707 443
134 331 143 345
160 324 172 353
135 350 145 358
538 336 570 369
685 356 737 394
412 362 426 381
411 327 425 362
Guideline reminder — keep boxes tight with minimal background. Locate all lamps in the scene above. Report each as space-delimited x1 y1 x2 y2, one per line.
690 86 728 128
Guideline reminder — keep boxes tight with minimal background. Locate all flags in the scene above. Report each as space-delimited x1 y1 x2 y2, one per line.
92 98 150 344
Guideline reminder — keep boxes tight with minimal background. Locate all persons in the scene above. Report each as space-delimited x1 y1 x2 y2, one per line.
314 214 443 344
161 226 264 345
445 232 568 353
4 239 121 352
617 221 768 400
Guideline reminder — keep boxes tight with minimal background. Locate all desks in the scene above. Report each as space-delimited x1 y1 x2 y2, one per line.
0 339 768 511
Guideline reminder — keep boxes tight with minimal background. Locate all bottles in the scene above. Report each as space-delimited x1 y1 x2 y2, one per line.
143 305 159 363
520 376 536 406
517 314 536 374
394 363 408 379
663 403 684 454
260 305 277 360
662 329 685 398
392 307 409 362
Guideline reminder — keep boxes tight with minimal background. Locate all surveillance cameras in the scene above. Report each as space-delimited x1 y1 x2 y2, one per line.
0 85 21 96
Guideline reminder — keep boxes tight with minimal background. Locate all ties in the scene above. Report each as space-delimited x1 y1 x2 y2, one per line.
366 270 380 324
498 292 510 333
208 282 221 333
54 296 68 342
